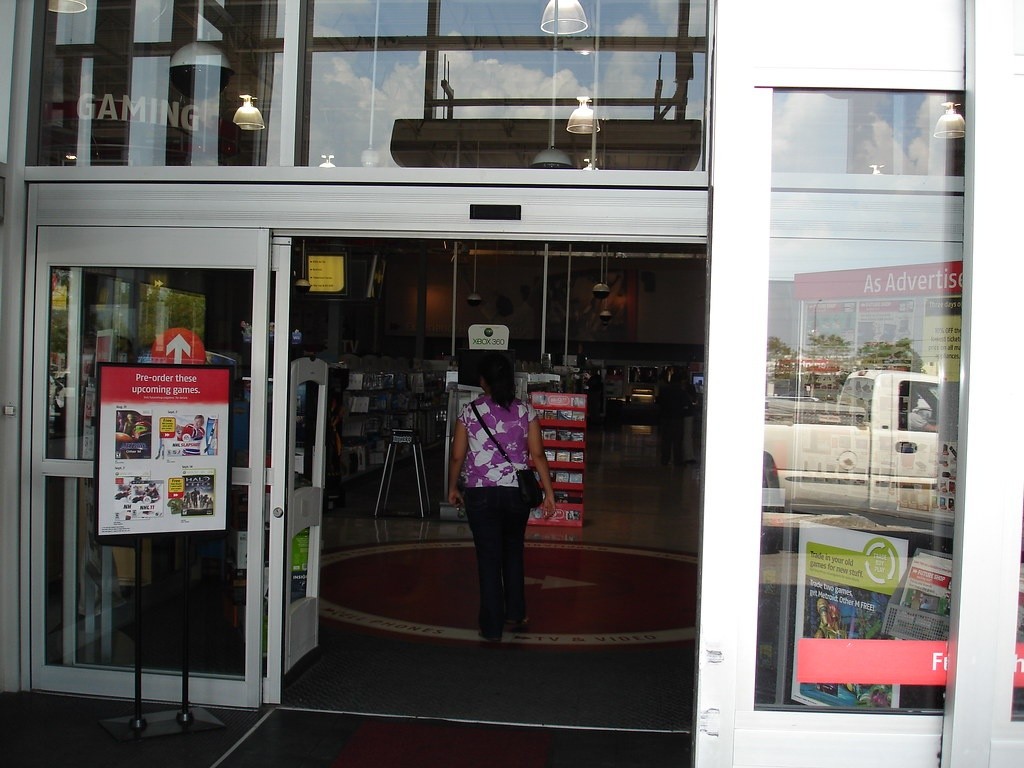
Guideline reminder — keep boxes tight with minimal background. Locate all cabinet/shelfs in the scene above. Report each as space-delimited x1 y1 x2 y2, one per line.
527 391 588 526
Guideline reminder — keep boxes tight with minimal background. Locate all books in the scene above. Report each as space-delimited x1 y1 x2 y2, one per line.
792 519 907 707
900 553 953 615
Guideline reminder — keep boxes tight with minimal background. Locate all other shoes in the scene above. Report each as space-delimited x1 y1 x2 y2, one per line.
504 614 530 624
478 630 502 641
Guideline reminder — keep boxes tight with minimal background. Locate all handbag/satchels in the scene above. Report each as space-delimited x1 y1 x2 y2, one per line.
335 432 341 456
515 469 543 507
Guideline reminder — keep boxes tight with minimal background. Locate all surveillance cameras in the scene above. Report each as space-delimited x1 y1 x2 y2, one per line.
169 41 234 101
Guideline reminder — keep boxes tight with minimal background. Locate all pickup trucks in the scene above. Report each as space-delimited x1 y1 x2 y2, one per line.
761 369 937 555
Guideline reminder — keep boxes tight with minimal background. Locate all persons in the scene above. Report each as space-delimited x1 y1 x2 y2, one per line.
122 414 135 435
177 415 205 456
184 488 213 509
133 482 160 502
907 390 939 432
563 367 603 429
448 353 556 640
655 373 694 468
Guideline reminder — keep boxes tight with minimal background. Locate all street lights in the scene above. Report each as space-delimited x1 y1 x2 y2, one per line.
808 299 824 397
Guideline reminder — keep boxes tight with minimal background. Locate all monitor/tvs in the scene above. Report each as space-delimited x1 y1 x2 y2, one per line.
691 372 704 386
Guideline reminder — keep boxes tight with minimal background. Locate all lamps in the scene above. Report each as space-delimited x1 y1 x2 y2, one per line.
233 85 265 129
567 85 600 134
581 151 599 171
66 150 76 160
933 92 965 139
540 0 588 34
